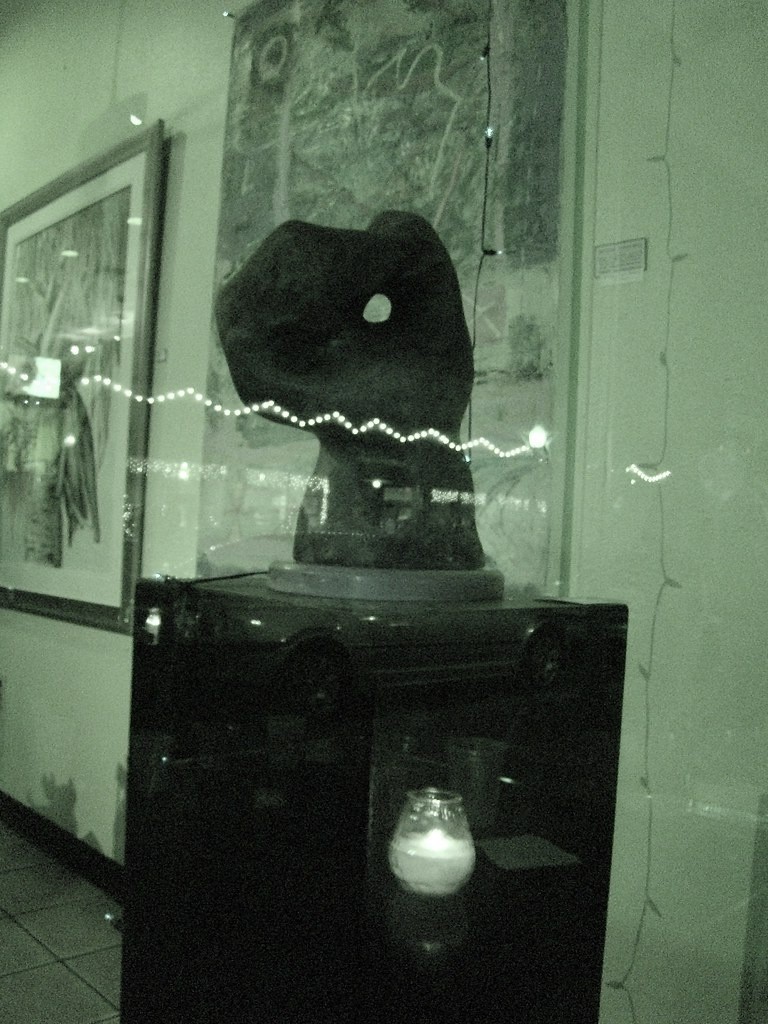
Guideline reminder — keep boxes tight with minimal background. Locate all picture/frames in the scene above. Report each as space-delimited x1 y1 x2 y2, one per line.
1 118 164 634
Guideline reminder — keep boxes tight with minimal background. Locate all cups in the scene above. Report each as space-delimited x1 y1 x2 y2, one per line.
388 786 475 898
